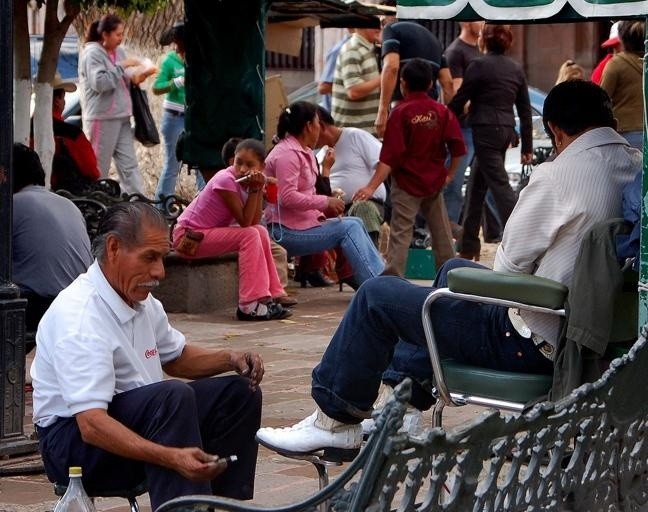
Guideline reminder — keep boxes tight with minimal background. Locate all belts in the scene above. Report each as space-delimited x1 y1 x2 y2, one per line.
164 108 185 118
508 307 556 361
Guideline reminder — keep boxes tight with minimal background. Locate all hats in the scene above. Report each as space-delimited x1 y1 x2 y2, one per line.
31 73 77 94
600 21 625 48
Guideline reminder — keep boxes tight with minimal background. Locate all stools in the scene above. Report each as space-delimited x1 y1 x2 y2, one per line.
54 481 152 512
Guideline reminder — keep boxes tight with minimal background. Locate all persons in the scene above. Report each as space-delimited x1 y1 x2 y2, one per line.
12 141 96 356
31 202 264 512
150 20 208 214
266 99 404 290
76 14 147 196
172 137 297 322
308 103 389 251
254 78 643 462
322 2 645 281
31 73 101 180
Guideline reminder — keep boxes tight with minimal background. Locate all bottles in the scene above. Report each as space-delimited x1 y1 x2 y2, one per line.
55 463 99 512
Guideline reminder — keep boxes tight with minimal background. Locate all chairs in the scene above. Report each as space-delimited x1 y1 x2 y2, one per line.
421 216 641 512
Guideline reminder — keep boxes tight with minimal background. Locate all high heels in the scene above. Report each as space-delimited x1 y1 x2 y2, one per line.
455 241 481 261
298 264 335 288
338 275 359 293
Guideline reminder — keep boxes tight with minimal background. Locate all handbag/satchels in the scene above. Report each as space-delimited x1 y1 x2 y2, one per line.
516 162 533 199
265 182 279 202
129 78 159 147
175 228 204 257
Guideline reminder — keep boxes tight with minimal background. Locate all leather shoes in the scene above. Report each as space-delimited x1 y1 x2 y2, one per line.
361 412 422 440
236 296 298 322
254 409 364 463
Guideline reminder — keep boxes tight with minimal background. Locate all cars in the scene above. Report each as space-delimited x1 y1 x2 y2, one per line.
28 33 82 128
461 82 554 198
